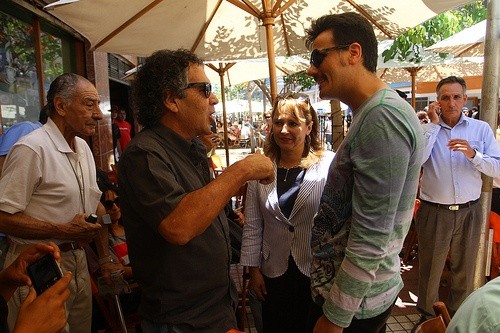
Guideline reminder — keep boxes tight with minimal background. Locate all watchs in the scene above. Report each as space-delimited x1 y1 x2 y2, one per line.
98 255 114 265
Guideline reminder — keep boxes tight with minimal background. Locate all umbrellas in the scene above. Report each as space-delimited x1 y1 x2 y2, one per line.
427 13 488 59
40 0 482 140
212 96 273 128
122 52 313 174
363 37 471 124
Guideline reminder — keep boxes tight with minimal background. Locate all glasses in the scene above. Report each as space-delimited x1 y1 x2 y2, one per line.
309 44 352 67
103 197 120 209
181 80 212 99
276 92 311 111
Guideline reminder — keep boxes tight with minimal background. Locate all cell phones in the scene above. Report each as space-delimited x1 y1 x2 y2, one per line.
26 251 63 297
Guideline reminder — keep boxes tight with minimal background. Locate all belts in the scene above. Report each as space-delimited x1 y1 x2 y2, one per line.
58 240 82 252
421 198 478 211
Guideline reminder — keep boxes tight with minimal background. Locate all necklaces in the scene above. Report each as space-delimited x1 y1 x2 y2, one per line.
278 160 308 182
279 157 302 164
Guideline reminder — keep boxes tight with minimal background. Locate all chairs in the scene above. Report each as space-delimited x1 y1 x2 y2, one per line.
234 266 251 333
488 210 500 281
238 137 251 149
414 300 452 333
404 198 421 264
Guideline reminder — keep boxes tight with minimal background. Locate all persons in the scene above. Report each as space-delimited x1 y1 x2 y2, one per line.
1 9 500 333
